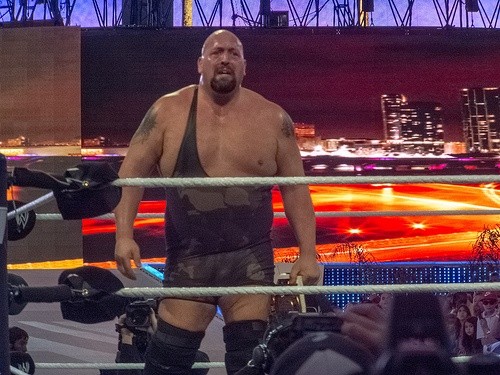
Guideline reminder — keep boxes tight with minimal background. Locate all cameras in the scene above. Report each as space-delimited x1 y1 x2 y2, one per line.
252 313 343 375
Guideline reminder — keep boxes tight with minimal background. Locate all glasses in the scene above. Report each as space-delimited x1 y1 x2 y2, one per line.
482 301 497 305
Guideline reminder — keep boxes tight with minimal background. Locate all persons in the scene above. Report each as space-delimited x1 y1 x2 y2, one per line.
115 29 321 375
9 326 28 353
99 274 500 375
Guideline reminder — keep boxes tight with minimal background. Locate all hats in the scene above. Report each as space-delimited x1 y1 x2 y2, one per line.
479 292 498 301
